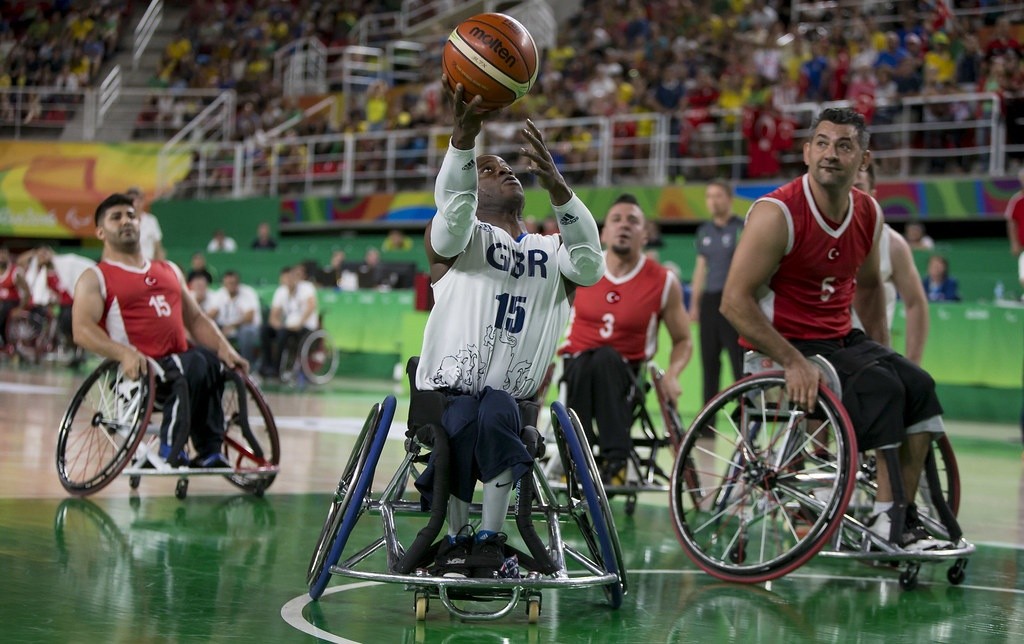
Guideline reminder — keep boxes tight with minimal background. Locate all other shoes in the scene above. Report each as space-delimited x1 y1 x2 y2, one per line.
195 454 231 468
159 444 189 466
597 456 627 486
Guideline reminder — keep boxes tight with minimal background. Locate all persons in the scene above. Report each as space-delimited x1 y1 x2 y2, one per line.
1003 169 1024 284
905 222 935 247
188 223 413 381
127 189 164 259
688 179 744 438
1 0 1024 194
558 193 692 485
416 74 608 586
720 108 958 552
0 245 98 369
923 256 958 299
72 193 250 468
787 165 930 508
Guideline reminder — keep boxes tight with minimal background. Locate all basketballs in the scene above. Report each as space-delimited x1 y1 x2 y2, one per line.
442 12 541 113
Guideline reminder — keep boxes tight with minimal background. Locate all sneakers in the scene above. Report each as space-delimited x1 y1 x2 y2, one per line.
473 531 508 578
434 524 476 579
863 503 955 550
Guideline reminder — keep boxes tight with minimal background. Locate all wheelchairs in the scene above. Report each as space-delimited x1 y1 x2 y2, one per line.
552 366 706 519
252 311 339 386
306 356 631 624
668 352 976 591
56 356 282 498
